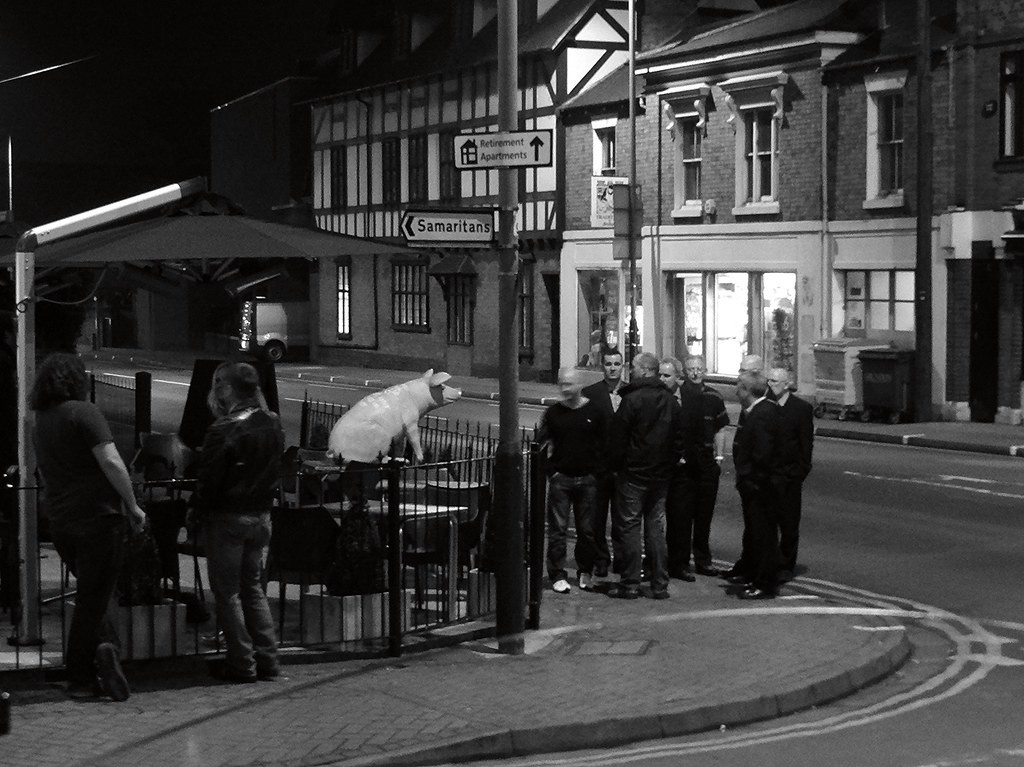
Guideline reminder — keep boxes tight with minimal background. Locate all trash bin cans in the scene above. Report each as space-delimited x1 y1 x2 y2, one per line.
856 346 917 425
811 335 889 422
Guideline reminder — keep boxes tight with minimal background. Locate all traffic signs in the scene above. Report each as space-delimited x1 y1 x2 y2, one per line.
454 130 553 170
399 207 500 247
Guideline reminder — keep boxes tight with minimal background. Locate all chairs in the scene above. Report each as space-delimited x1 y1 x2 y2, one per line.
0 433 493 632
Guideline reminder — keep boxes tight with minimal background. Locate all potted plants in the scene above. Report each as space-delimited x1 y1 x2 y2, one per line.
468 494 531 621
65 500 187 660
303 492 411 644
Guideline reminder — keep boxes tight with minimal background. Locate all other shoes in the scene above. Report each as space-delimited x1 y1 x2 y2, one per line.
93 642 130 701
606 584 638 600
669 566 695 581
695 563 718 575
66 680 106 698
211 659 280 682
641 583 669 599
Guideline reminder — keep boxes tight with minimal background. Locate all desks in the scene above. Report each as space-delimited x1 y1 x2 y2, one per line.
301 500 469 623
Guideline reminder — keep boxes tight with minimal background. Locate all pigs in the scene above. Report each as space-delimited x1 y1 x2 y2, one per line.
326 368 463 468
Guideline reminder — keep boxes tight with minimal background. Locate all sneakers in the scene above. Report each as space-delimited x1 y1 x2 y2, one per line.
578 572 607 591
552 578 571 592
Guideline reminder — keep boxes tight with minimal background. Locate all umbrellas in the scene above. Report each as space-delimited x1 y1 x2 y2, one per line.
0 172 434 650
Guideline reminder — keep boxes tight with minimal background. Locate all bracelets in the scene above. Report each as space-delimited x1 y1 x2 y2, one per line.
714 456 725 461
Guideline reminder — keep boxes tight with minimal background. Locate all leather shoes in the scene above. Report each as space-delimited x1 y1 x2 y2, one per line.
719 567 775 599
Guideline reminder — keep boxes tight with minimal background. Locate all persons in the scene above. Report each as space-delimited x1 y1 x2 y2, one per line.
579 349 633 590
535 364 610 593
718 352 768 577
604 352 684 600
725 369 784 599
657 357 698 581
28 351 148 701
684 353 730 577
184 359 287 685
762 367 814 572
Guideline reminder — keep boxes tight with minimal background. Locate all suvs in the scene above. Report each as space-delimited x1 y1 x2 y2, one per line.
238 294 311 363
